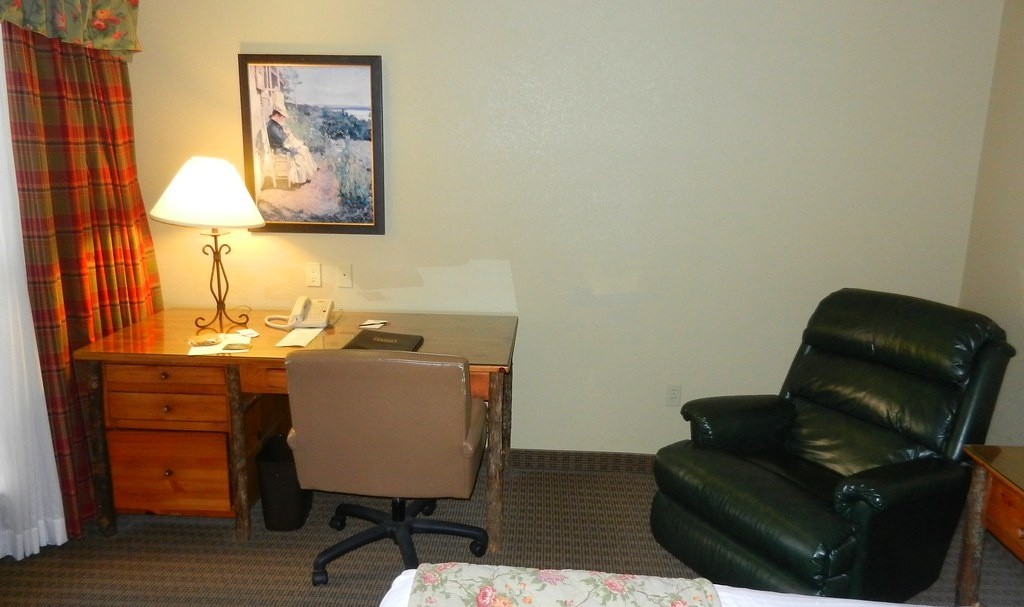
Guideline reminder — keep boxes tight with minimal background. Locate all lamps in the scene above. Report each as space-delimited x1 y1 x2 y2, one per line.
150 157 265 328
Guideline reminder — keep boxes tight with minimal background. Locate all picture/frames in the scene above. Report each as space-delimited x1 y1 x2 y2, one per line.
238 55 386 235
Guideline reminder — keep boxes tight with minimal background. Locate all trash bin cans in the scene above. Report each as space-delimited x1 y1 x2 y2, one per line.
257 433 315 532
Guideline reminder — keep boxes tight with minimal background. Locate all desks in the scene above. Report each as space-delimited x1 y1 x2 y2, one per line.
74 309 518 542
955 444 1024 607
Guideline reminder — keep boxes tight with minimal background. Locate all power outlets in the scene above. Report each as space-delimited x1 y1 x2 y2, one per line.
305 262 322 287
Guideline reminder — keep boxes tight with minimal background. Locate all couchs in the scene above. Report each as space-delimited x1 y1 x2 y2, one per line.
651 287 1017 602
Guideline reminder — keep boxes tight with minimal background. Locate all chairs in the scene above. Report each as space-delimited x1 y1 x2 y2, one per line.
286 349 489 585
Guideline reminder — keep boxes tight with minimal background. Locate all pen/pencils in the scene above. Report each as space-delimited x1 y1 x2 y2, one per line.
358 322 388 326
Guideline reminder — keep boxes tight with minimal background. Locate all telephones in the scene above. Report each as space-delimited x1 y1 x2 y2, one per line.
288 296 335 329
